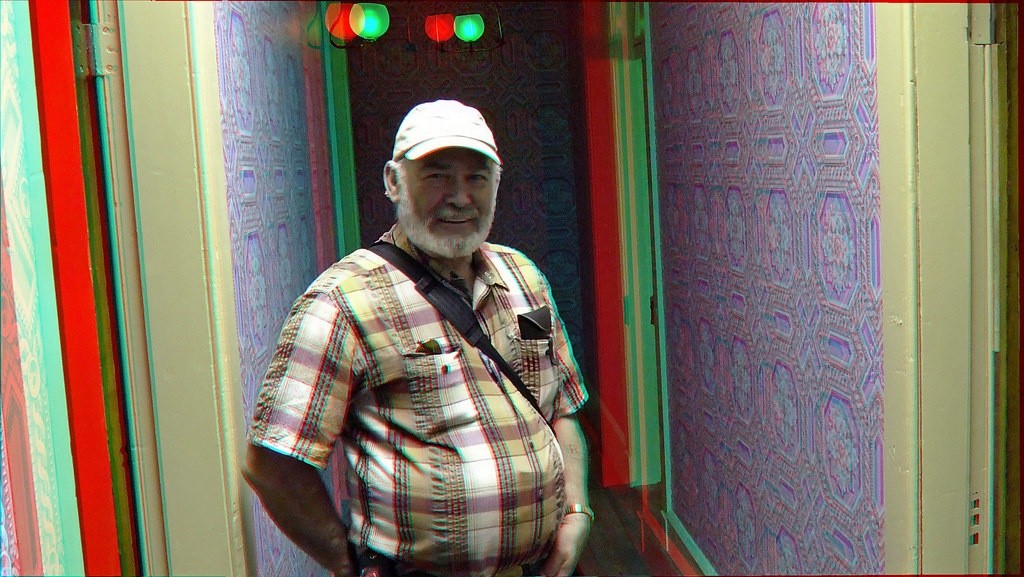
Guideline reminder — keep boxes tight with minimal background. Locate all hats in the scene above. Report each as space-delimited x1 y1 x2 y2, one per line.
393 99 501 166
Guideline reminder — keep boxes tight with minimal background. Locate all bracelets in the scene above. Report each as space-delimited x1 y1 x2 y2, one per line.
564 502 594 521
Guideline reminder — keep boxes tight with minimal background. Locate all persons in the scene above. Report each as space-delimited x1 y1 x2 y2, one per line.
239 99 595 577
